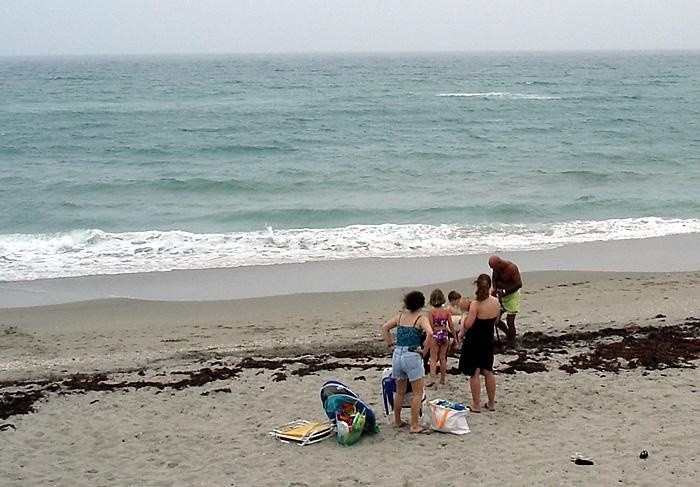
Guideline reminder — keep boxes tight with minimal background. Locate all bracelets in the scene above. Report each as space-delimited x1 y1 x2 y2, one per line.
503 288 506 295
387 343 394 348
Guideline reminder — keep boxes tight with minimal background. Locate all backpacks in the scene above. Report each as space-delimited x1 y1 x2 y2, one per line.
381 368 426 416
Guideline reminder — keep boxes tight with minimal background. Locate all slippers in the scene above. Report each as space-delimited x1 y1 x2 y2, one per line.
393 420 432 434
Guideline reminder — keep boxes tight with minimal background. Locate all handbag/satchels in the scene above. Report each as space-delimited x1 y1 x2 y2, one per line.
423 399 472 435
334 404 367 446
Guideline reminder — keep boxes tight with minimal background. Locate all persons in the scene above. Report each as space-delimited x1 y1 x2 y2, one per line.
457 301 473 344
382 289 434 437
489 255 523 346
428 287 459 388
444 290 461 357
457 272 502 413
